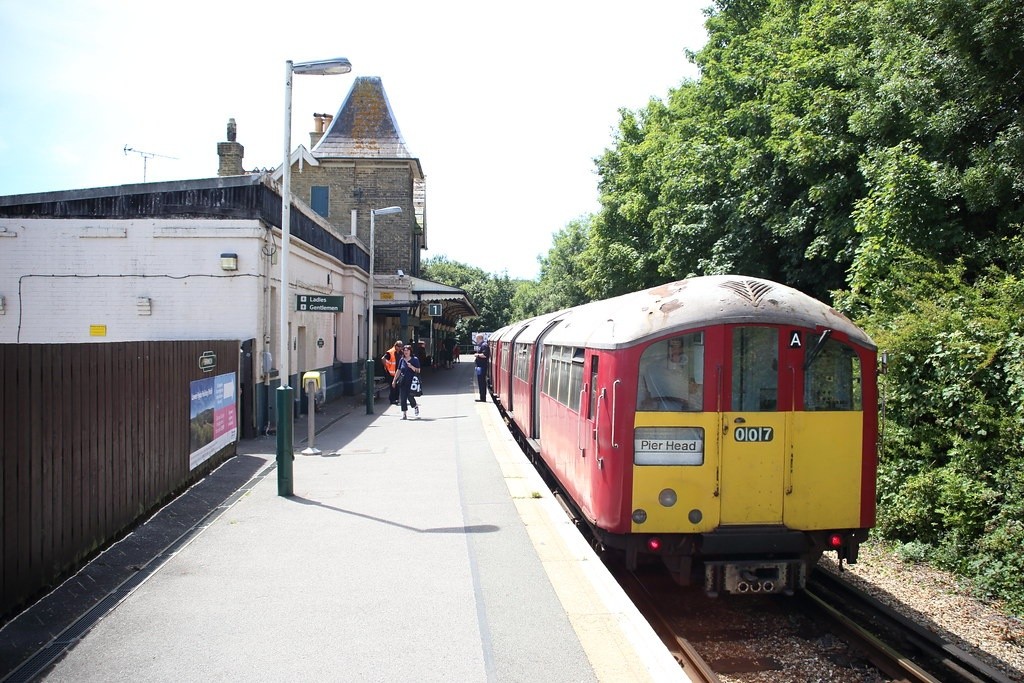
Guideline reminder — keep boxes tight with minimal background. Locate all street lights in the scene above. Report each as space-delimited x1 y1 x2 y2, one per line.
275 58 353 494
366 205 402 412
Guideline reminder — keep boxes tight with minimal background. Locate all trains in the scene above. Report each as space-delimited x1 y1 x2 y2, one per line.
488 275 879 602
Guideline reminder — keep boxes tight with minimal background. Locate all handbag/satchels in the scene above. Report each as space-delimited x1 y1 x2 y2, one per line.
396 372 405 386
410 368 423 397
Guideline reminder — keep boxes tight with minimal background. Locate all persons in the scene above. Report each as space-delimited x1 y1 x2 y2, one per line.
437 334 461 369
381 340 403 405
474 334 490 402
392 345 421 420
407 339 421 360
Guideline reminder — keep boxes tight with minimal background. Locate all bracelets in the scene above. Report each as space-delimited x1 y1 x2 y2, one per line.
478 355 479 358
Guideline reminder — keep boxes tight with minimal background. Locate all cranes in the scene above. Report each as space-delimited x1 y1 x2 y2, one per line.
120 145 181 183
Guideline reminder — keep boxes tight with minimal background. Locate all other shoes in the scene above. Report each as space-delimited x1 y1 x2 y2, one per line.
475 399 486 402
400 414 407 420
390 401 398 405
415 407 419 416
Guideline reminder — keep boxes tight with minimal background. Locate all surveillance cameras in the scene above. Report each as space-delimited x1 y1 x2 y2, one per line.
398 270 404 277
